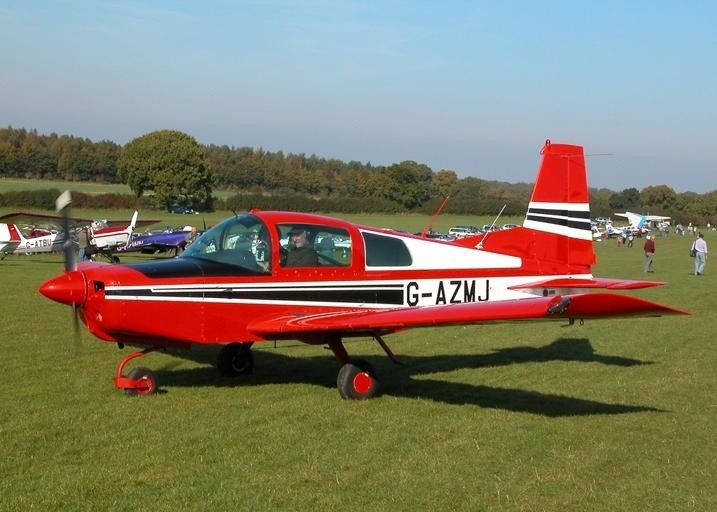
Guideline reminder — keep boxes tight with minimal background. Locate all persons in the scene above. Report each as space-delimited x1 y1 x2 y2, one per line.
601 220 717 249
643 235 656 273
279 224 319 267
691 234 708 276
318 233 335 253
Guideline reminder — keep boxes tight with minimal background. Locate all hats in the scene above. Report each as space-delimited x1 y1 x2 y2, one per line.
286 224 311 234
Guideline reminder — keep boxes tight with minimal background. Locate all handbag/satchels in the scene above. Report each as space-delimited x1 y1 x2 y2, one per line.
689 248 697 257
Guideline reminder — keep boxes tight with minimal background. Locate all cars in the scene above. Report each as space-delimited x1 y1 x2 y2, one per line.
169 206 193 215
413 223 521 241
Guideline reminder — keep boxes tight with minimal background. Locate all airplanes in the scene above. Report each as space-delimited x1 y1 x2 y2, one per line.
590 211 670 241
37 142 698 402
0 210 139 264
107 217 206 256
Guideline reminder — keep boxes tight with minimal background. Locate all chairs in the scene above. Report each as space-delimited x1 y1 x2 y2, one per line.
318 237 335 266
